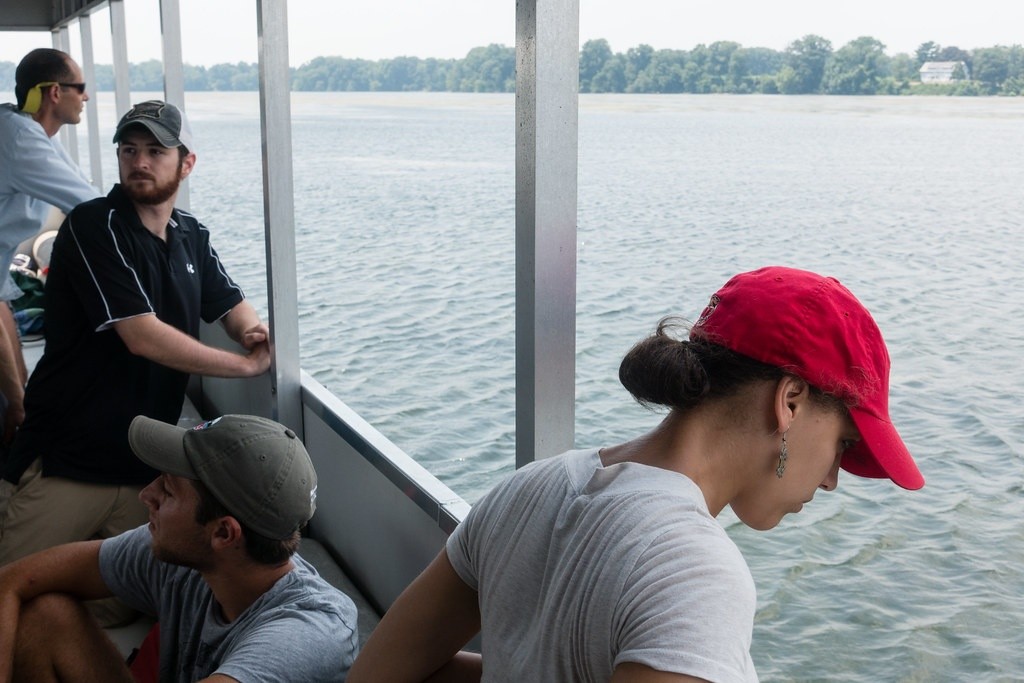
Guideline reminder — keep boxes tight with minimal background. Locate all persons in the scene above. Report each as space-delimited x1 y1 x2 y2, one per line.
24 99 272 487
355 266 926 683
0 48 107 338
0 415 360 683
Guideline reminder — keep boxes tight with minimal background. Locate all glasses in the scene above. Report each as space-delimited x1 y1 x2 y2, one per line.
56 81 86 94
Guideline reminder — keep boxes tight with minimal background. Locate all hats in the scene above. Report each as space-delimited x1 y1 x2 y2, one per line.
686 265 926 492
125 412 321 542
112 98 194 154
